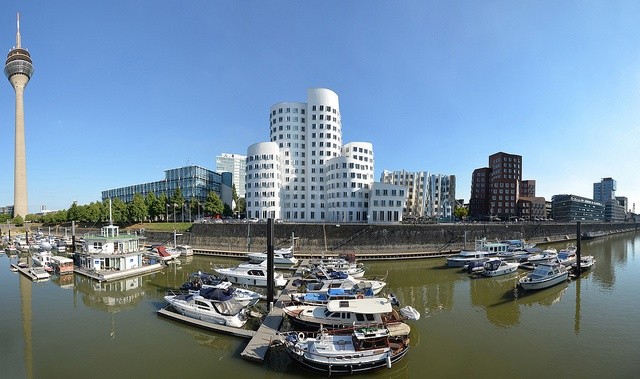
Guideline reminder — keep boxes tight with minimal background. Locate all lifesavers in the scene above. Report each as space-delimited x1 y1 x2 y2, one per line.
298 332 305 339
356 294 364 299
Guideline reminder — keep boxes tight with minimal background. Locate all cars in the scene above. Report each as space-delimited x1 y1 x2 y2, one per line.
199 217 290 224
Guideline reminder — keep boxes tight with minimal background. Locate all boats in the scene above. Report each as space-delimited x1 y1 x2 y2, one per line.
301 265 365 279
572 255 596 274
446 242 509 267
296 267 386 296
482 259 520 277
0 230 195 277
518 258 569 290
162 287 262 328
497 244 536 262
279 296 421 330
178 271 263 308
464 257 507 273
276 322 411 376
528 248 558 265
285 282 400 306
315 259 364 269
499 239 539 250
246 245 299 270
208 259 288 287
548 247 578 267
